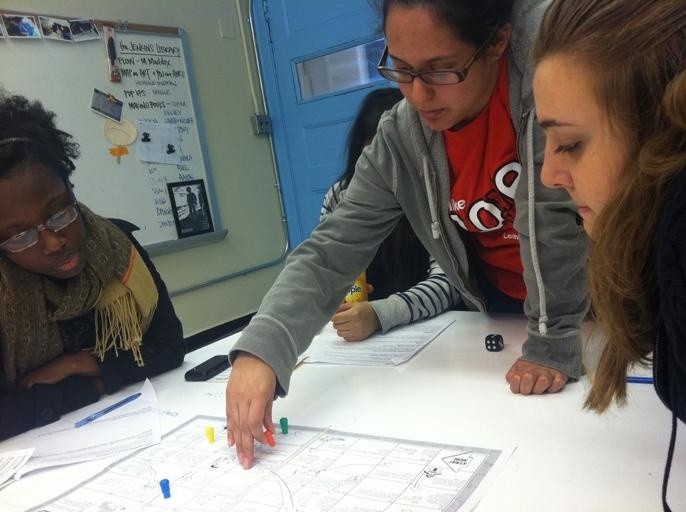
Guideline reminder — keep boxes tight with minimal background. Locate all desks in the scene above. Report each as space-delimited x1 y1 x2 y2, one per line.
0 310 686 512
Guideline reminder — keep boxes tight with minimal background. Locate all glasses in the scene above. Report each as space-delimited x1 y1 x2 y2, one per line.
376 18 506 88
1 174 83 254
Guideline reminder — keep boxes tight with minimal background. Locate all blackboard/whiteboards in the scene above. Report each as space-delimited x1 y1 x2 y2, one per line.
1 9 230 260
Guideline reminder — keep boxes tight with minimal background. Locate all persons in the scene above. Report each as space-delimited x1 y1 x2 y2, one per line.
523 0 685 433
106 30 118 74
311 85 466 344
186 187 199 215
223 2 600 473
11 17 38 36
0 89 190 444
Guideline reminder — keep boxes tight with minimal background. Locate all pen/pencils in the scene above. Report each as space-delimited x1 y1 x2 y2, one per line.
627 377 654 383
75 393 144 429
